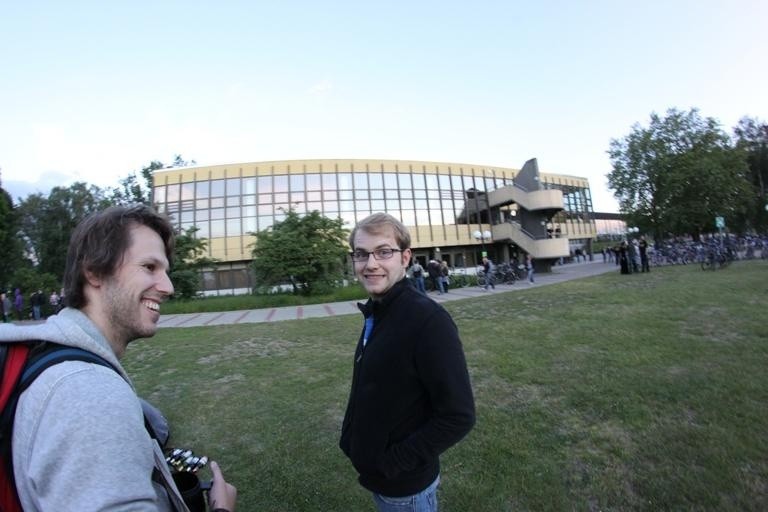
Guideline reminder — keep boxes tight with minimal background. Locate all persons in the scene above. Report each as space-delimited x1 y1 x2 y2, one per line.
339 212 476 512
575 247 587 262
0 203 237 512
409 257 450 295
481 256 497 291
601 235 650 274
0 288 66 323
526 254 537 285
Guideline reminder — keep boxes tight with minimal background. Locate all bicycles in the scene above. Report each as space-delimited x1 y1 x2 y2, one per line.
651 236 768 271
449 262 520 290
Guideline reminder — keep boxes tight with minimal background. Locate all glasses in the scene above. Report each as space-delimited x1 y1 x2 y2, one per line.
350 248 403 261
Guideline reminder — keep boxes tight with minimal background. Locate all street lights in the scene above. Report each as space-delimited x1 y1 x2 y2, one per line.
473 230 492 256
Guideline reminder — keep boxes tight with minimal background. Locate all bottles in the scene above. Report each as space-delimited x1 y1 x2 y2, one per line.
165 448 208 477
